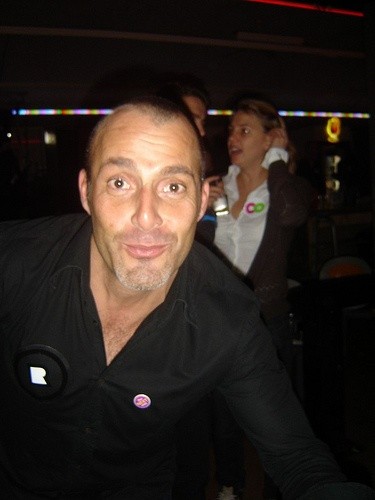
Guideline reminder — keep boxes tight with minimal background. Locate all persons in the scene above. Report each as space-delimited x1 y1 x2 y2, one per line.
210 98 316 500
1 93 375 500
158 82 226 500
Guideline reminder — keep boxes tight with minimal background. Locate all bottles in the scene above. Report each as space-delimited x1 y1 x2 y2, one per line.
212 176 229 216
